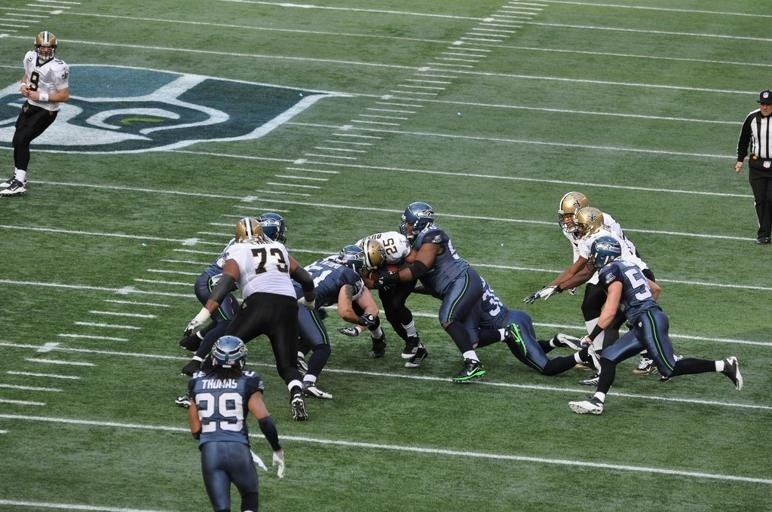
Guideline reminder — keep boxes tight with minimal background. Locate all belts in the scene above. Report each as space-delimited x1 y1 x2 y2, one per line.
750 154 772 169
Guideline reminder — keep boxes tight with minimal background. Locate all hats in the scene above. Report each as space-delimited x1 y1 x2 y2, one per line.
757 90 772 105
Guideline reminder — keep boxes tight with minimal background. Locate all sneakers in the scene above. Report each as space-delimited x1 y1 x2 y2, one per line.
757 237 771 244
720 356 743 392
0 173 27 188
0 179 25 196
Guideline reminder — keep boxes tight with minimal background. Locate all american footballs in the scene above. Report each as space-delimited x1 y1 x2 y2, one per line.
369 265 399 282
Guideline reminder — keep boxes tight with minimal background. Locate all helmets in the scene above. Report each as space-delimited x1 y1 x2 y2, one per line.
400 202 434 243
558 192 589 233
34 31 57 61
590 237 621 271
359 239 386 271
338 244 366 273
236 217 263 242
573 207 604 240
258 213 287 245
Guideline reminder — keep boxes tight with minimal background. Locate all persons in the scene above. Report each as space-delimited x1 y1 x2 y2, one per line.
522 192 745 416
0 29 72 198
734 86 772 245
171 190 602 416
186 333 288 512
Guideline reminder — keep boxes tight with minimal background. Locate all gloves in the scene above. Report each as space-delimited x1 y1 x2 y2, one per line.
183 306 212 337
522 285 563 304
568 287 576 296
337 327 361 336
298 296 316 311
374 270 400 295
358 266 372 280
273 451 285 479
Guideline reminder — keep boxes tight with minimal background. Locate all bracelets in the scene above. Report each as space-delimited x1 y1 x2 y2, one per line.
19 82 26 87
38 92 48 102
